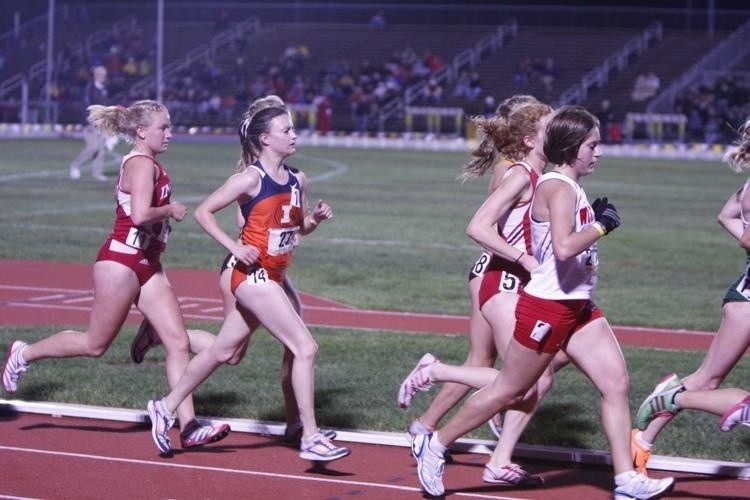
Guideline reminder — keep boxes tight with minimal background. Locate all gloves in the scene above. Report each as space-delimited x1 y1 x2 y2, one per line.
592 196 615 220
598 203 621 234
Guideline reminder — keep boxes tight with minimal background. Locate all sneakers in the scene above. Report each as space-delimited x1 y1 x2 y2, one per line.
488 413 502 438
95 175 107 181
130 318 156 365
1 337 32 394
283 426 336 446
397 352 439 408
405 418 431 441
614 473 674 500
635 373 686 432
298 434 351 461
180 423 230 447
482 464 545 487
411 431 446 496
69 168 81 179
145 399 175 454
631 427 651 477
719 394 750 430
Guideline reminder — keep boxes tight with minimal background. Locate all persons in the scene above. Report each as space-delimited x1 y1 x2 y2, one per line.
68 66 113 182
636 371 750 432
513 55 557 90
674 75 748 143
1 99 231 449
628 119 750 477
130 94 337 447
398 103 555 489
411 103 675 500
146 106 351 462
485 96 496 114
592 99 611 138
367 8 386 30
453 70 482 102
405 95 570 463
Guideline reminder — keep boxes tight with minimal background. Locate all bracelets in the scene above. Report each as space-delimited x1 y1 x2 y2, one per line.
514 251 526 265
592 222 606 238
